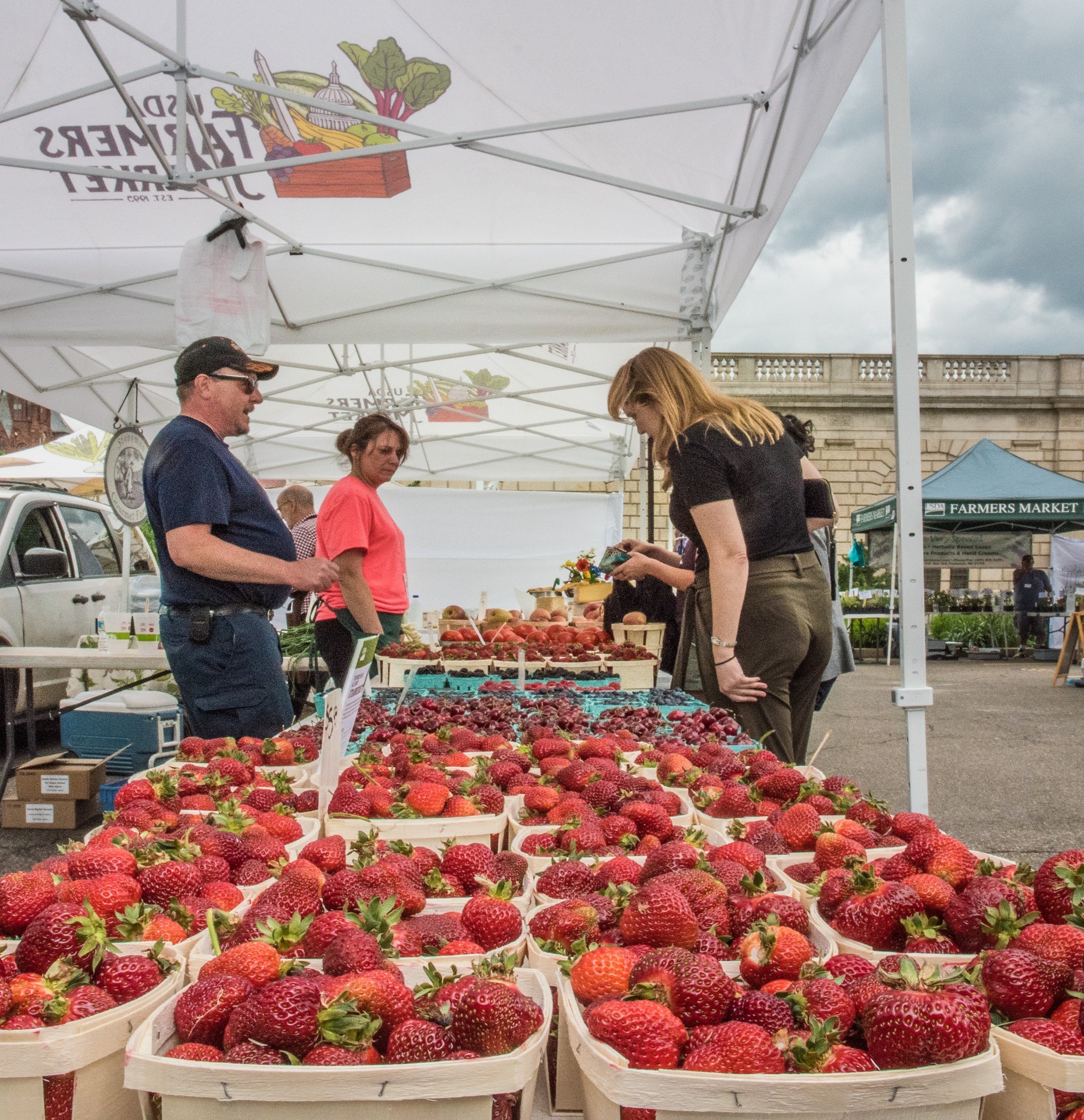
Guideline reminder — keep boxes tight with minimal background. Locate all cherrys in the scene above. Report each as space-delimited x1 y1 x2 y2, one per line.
279 695 754 745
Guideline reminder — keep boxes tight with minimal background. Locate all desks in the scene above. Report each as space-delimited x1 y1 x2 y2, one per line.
841 612 899 663
924 609 1008 661
0 644 328 801
1007 610 1075 660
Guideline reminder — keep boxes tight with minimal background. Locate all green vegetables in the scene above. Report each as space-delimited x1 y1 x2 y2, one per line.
277 622 316 697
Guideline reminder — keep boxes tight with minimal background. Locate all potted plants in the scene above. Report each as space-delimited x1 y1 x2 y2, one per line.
838 585 1084 663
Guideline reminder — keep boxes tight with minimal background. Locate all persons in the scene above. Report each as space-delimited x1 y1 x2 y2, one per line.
308 414 407 688
606 349 832 769
599 411 856 711
1012 555 1055 658
144 338 340 742
276 485 324 634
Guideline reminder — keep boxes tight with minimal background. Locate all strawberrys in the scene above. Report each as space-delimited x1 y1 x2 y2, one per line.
379 640 660 662
0 728 1084 1120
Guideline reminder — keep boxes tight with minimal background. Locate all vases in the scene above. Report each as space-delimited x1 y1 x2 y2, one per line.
571 571 581 584
581 569 592 582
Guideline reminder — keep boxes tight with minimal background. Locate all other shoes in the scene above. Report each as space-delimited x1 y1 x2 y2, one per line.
1014 649 1025 657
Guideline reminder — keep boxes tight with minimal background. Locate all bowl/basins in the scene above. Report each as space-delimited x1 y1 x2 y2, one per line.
529 590 562 597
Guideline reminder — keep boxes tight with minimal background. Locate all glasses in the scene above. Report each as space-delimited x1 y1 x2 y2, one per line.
208 371 257 394
275 500 292 514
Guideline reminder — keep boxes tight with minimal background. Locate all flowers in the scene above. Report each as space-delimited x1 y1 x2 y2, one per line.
559 547 602 579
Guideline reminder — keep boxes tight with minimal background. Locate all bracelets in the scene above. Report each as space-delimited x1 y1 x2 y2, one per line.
712 655 735 667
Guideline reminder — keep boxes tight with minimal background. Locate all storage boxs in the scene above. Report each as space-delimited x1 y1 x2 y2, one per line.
0 620 1084 1120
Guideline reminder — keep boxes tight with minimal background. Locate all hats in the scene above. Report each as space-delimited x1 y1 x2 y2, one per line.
1023 555 1035 564
174 336 279 386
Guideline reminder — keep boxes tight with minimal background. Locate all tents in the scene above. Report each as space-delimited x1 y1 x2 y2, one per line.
0 0 947 825
847 436 1084 671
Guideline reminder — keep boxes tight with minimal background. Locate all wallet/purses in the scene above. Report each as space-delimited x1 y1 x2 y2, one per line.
598 547 632 573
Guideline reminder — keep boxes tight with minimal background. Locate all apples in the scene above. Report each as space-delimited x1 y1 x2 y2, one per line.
623 612 646 625
443 602 604 626
440 624 612 645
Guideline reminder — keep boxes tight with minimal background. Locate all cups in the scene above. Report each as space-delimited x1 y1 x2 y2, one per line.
104 612 133 653
132 612 161 650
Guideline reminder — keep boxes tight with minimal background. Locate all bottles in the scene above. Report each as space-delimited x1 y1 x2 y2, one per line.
97 605 115 653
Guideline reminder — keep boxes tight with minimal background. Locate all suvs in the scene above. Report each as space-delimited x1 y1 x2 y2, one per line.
0 477 170 744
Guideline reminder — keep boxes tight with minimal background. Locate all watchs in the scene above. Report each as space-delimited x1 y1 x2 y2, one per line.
710 636 737 648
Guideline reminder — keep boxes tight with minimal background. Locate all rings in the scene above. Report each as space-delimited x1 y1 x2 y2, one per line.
621 571 627 579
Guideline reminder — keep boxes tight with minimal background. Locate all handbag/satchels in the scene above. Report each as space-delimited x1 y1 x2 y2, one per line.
600 573 680 676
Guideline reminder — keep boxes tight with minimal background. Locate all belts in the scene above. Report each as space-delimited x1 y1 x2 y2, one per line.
160 602 275 622
